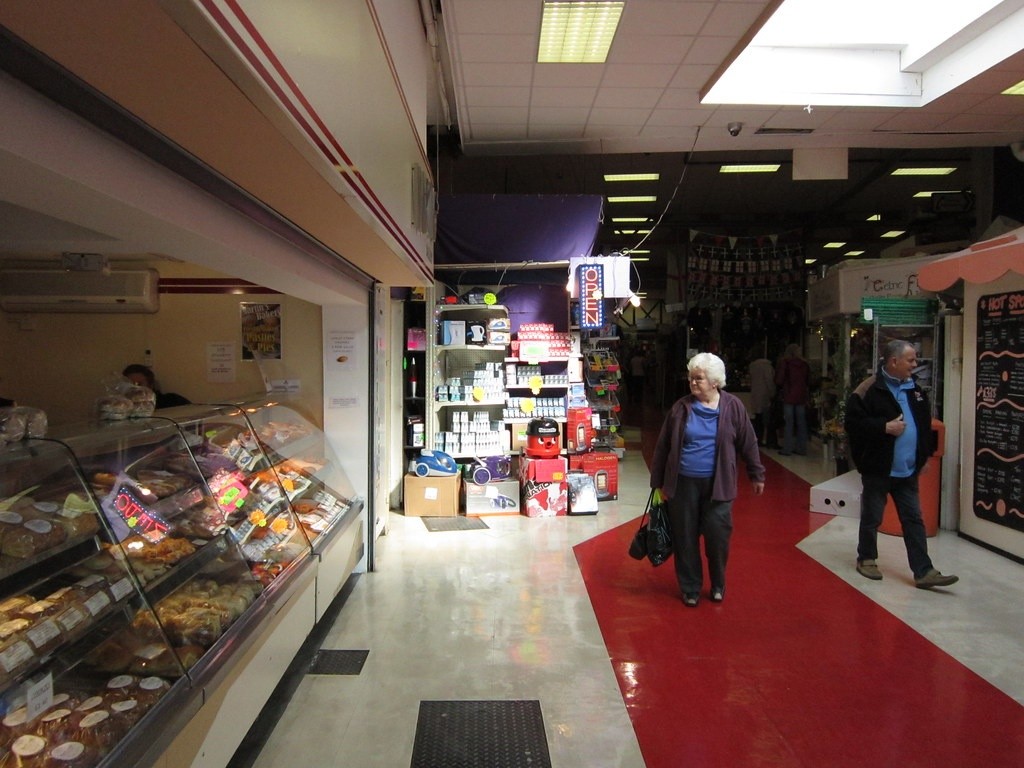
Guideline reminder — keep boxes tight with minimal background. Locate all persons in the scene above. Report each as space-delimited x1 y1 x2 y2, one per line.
844 340 959 588
774 344 810 455
749 353 783 450
125 365 189 410
650 352 766 607
618 344 648 403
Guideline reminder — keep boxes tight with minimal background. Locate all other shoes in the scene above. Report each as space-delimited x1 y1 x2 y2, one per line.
709 580 726 600
778 451 792 456
915 568 959 590
683 591 699 605
792 450 806 456
856 558 883 580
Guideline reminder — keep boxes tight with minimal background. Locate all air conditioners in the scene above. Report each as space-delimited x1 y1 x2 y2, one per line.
0 268 158 315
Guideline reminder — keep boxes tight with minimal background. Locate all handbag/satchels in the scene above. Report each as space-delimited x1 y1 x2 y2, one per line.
628 488 674 567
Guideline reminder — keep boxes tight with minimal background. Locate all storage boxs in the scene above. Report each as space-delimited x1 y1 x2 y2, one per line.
402 287 618 517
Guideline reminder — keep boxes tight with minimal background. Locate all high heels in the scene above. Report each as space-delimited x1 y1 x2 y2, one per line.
766 439 783 450
758 441 765 447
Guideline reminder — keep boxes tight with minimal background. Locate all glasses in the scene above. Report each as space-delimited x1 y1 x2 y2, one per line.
687 376 703 384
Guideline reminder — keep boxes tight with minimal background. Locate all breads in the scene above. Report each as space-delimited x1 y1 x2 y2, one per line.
96 391 156 419
0 495 256 768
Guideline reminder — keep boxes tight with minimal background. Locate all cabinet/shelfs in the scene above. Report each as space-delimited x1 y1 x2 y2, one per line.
0 396 365 768
866 315 939 419
403 284 625 460
